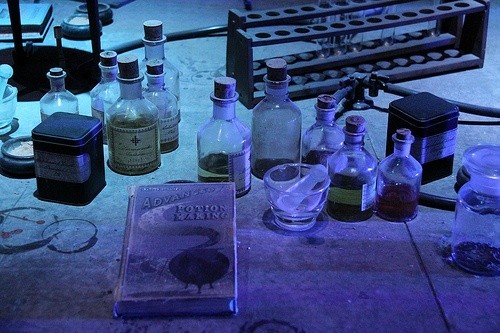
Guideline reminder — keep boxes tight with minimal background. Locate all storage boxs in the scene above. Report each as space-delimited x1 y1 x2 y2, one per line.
386 91 460 185
32 112 105 204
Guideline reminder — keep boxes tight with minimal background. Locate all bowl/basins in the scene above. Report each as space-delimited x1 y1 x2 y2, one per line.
0 136 35 173
0 84 20 128
60 14 103 38
263 161 330 231
74 2 113 25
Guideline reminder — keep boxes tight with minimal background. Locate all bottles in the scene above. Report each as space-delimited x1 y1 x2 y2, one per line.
90 51 119 146
38 67 79 121
375 130 422 223
250 59 302 180
195 76 251 199
104 54 161 177
301 95 343 165
138 19 182 124
327 116 379 223
140 57 180 154
450 144 499 277
